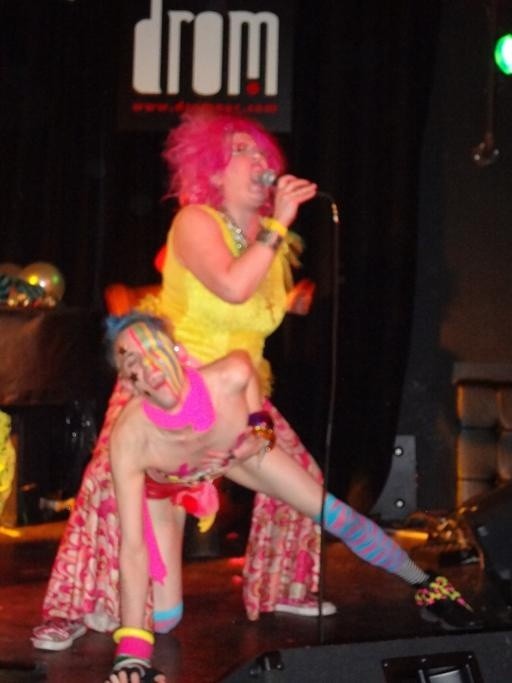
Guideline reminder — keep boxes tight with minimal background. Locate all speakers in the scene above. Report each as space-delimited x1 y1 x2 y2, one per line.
463 479 512 608
221 631 512 683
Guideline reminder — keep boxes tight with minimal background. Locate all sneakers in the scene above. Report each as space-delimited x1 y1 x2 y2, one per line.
413 570 477 632
274 592 337 617
33 616 88 652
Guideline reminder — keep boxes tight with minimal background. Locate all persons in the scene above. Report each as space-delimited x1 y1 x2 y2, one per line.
27 111 479 683
0 260 77 558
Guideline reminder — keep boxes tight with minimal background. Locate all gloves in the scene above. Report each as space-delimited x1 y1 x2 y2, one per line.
104 651 165 682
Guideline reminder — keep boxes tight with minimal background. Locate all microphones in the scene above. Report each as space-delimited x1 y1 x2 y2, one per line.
259 169 334 201
